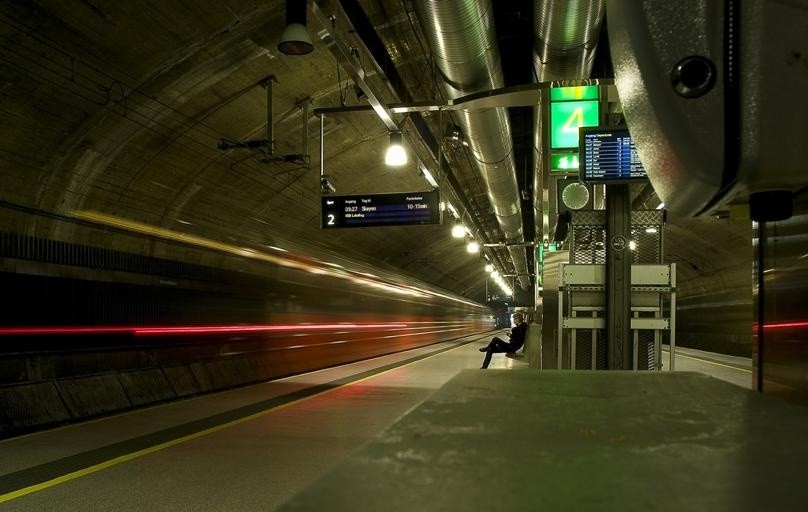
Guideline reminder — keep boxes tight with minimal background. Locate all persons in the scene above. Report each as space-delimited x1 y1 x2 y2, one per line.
479 313 527 369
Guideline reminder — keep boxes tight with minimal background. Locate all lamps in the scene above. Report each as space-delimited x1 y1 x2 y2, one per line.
384 131 407 166
451 219 513 297
277 0 313 55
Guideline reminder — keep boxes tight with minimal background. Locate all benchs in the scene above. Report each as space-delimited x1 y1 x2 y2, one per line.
506 326 530 363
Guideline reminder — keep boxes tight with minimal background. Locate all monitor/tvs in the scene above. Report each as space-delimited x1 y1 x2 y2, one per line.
578 126 650 185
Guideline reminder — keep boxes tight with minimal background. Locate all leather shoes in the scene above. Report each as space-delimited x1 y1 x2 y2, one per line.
479 346 487 352
